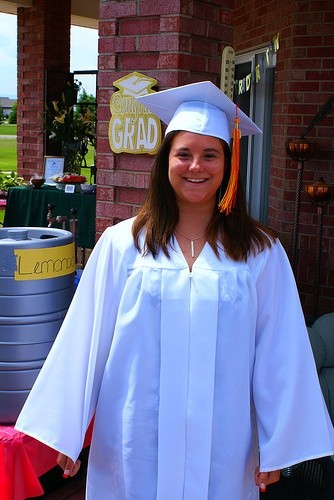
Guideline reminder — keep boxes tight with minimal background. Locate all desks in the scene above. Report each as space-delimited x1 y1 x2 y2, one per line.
0 413 96 500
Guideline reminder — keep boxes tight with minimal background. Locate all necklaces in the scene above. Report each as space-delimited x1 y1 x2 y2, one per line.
174 229 206 257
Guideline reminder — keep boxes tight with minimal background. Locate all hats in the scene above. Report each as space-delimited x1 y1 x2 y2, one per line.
135 82 263 146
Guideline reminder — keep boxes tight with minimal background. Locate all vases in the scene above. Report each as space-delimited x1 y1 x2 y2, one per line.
61 141 81 175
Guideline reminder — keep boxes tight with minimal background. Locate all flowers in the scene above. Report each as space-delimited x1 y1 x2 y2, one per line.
38 92 97 149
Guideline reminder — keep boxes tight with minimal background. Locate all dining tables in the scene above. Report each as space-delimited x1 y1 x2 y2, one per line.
3 184 96 249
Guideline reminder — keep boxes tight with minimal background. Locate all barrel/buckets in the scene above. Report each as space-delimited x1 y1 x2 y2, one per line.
0 227 76 426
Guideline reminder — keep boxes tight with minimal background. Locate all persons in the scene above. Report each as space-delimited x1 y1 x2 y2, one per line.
13 81 334 500
